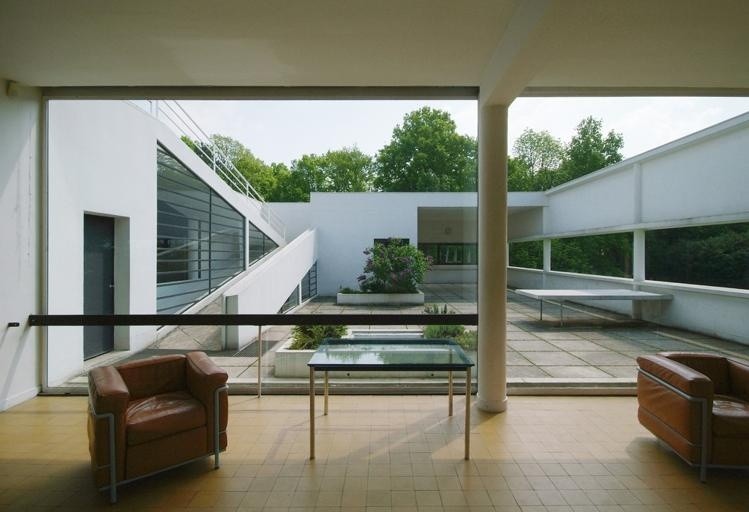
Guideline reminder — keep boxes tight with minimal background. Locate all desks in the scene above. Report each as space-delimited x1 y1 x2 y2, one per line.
515 289 673 329
307 337 473 461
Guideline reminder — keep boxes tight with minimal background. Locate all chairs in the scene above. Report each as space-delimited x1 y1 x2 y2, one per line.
88 350 227 505
635 351 749 482
